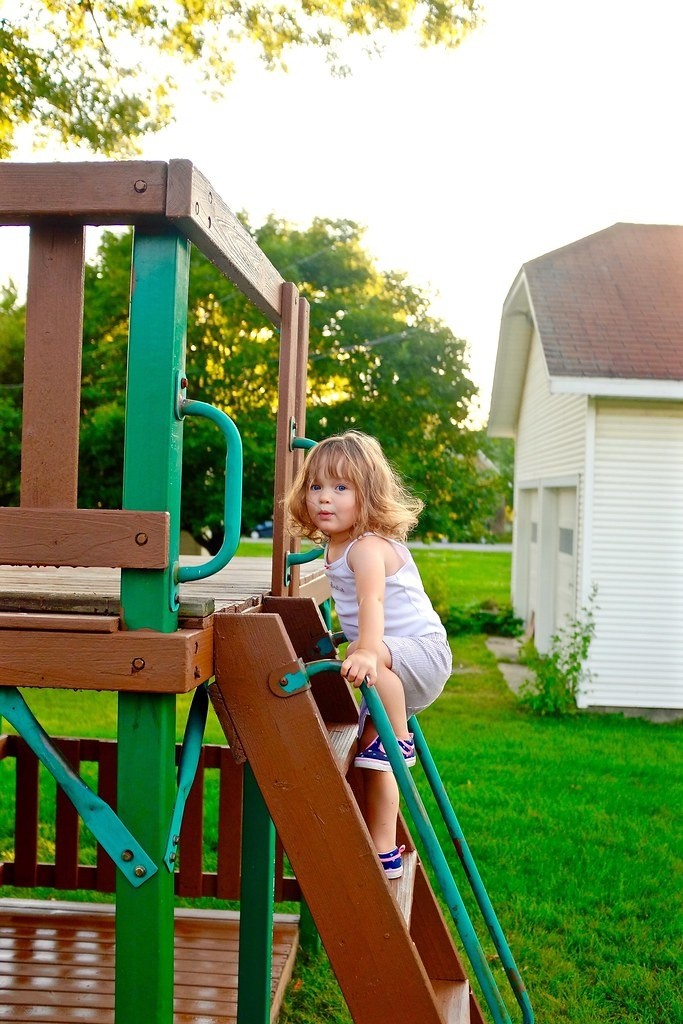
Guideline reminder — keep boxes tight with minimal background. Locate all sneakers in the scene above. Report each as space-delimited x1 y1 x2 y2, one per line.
353 732 417 773
377 845 406 881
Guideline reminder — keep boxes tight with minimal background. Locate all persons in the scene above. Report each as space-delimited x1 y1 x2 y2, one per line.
278 429 452 879
